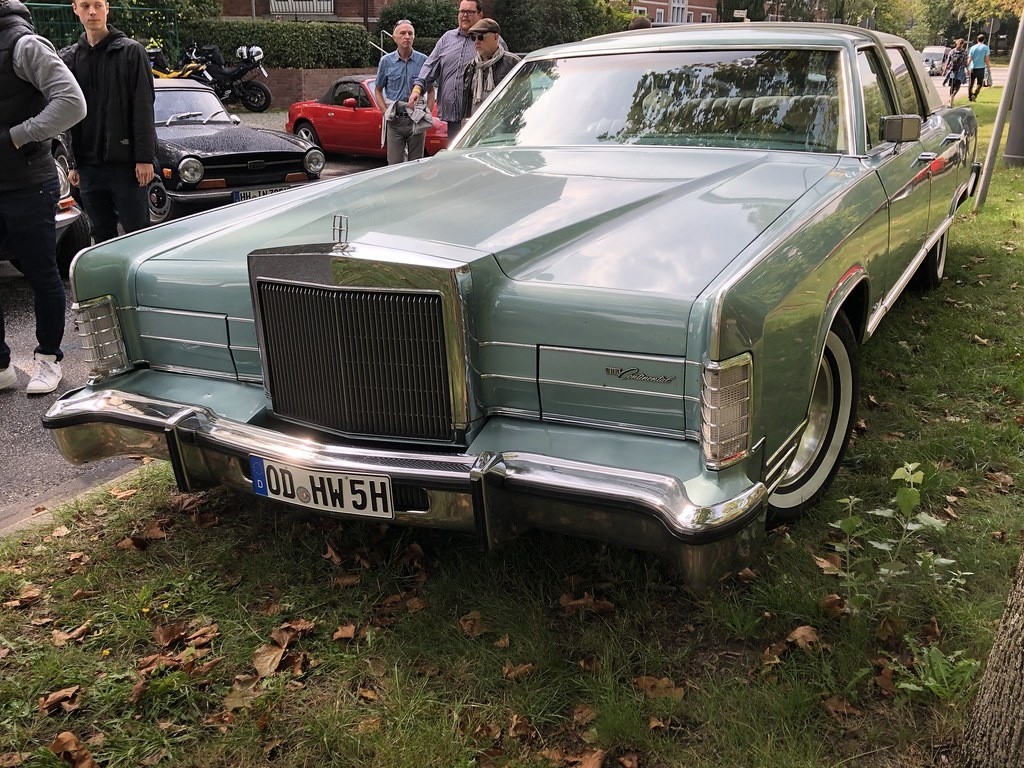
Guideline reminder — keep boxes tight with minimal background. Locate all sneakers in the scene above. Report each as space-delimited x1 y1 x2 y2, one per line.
0 360 18 390
26 353 63 394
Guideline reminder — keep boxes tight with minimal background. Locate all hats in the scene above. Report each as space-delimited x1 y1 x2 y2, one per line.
467 18 500 34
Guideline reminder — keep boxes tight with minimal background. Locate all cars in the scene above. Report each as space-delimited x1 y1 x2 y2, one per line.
49 78 327 246
285 73 448 164
41 21 984 595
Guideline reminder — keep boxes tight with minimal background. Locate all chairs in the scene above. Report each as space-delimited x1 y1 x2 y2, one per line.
610 95 839 143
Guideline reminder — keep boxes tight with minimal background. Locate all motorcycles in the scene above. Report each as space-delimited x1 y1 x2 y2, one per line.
142 33 272 113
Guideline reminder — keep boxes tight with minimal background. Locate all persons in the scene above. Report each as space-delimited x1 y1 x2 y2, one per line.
373 20 438 166
0 0 87 394
408 0 508 148
942 38 970 106
968 34 991 102
57 0 156 245
461 18 533 148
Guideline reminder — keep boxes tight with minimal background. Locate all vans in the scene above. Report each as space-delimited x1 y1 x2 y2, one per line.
922 46 952 75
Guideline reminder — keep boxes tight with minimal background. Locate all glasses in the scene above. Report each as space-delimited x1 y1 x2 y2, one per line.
393 20 412 29
457 9 480 14
471 34 495 41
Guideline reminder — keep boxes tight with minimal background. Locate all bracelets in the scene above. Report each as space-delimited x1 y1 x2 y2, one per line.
412 89 420 93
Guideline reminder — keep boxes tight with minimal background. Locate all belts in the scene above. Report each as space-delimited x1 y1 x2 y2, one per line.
394 112 412 118
